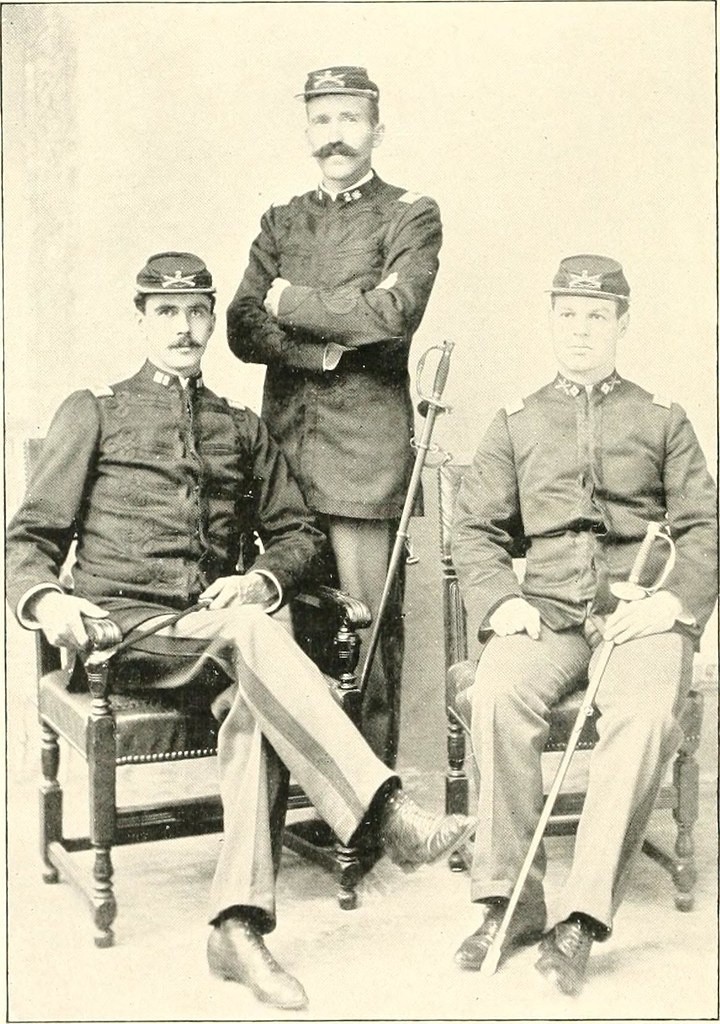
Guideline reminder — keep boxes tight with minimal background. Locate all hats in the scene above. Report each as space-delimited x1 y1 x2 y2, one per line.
294 66 378 101
551 256 629 300
136 252 216 295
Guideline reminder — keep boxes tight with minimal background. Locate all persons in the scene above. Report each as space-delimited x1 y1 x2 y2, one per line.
451 256 719 995
2 253 482 1008
226 68 443 771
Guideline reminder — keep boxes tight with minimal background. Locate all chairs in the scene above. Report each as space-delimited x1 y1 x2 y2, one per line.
437 463 704 913
23 429 372 949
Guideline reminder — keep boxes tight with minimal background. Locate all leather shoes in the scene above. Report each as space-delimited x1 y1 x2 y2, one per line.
536 916 593 999
458 895 547 969
206 911 306 1011
377 792 477 873
